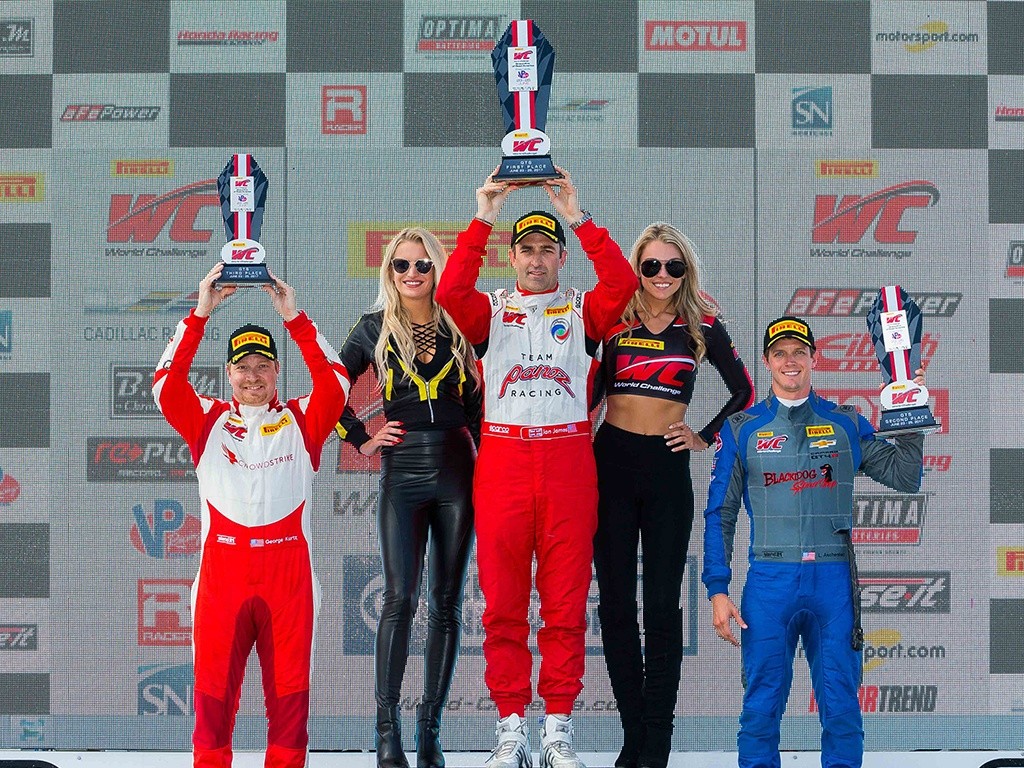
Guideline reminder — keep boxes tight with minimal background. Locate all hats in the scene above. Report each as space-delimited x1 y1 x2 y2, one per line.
511 211 566 248
763 316 815 354
228 323 277 364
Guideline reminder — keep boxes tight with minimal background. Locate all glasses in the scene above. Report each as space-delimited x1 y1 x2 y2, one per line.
638 259 687 278
390 258 434 275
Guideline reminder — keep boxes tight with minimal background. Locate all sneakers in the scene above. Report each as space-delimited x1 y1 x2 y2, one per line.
485 713 533 768
539 715 586 768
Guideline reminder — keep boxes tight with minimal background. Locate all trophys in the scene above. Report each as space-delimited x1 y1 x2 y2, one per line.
865 285 941 443
491 20 562 189
213 153 275 290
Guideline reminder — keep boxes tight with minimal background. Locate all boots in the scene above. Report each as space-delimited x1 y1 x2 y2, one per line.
375 705 409 768
598 604 643 768
639 606 683 768
414 704 445 768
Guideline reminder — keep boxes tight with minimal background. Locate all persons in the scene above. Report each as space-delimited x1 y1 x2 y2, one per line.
582 220 754 768
435 159 639 768
150 262 351 768
328 225 476 768
700 318 929 767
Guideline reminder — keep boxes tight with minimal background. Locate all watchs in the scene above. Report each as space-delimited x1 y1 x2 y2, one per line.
569 208 594 230
